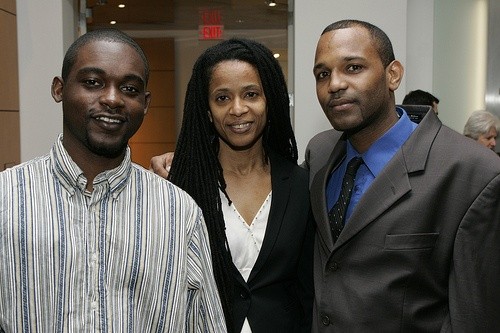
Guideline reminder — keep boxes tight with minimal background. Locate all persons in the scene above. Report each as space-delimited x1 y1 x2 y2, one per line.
0 30 228 333
150 20 500 333
402 90 439 121
463 110 500 152
163 37 314 333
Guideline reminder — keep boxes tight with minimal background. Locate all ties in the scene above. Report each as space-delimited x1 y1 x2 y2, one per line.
328 156 366 246
